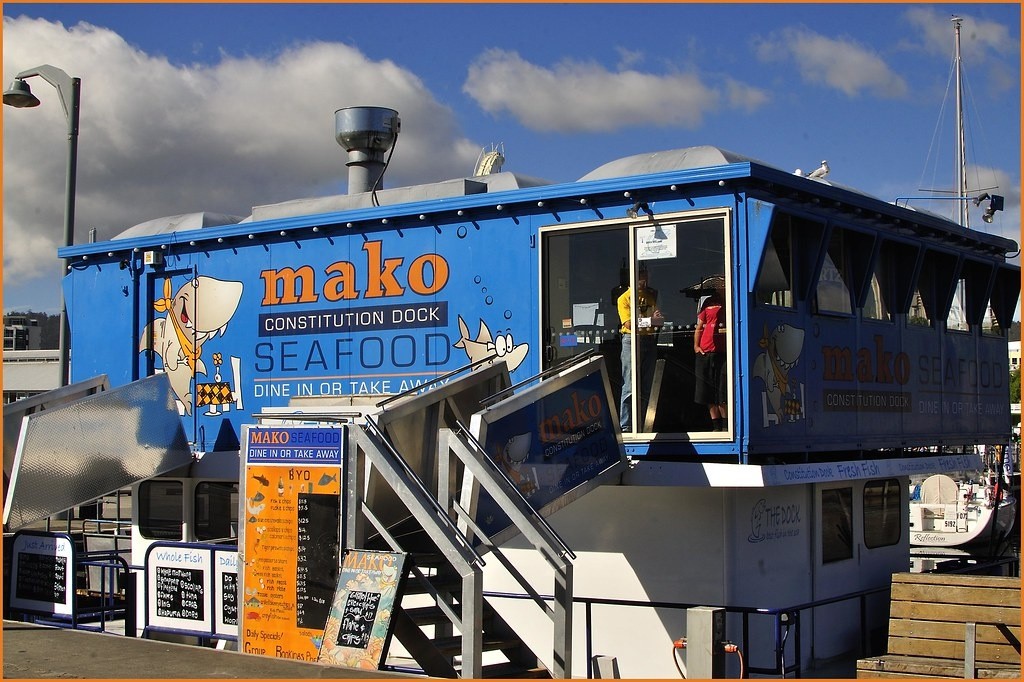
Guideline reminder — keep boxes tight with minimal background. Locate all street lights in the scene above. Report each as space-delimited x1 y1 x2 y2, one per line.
2 63 82 389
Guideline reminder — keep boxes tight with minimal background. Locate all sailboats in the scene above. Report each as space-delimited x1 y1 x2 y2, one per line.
895 14 1018 550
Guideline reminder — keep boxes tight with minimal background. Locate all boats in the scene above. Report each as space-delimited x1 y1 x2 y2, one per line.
5 104 1020 678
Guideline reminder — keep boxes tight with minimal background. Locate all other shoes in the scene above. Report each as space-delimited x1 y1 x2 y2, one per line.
713 424 729 432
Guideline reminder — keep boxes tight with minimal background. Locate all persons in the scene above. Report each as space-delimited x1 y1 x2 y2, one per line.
694 294 728 432
617 268 663 432
